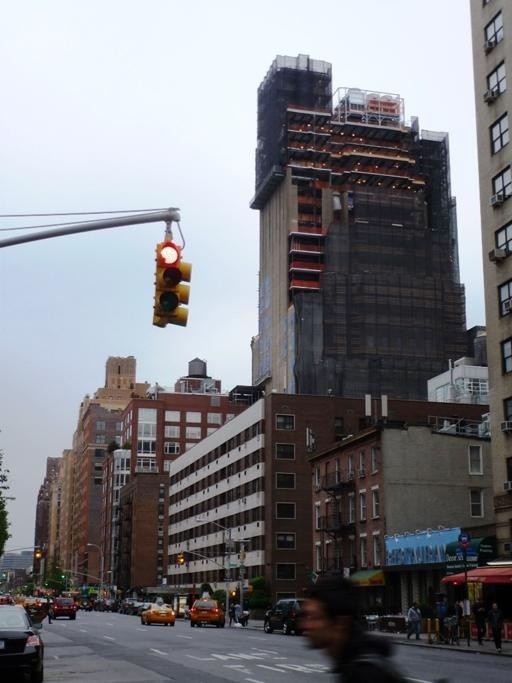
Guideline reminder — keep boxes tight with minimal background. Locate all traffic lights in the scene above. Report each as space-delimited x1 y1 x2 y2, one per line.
178 553 184 565
232 589 240 600
153 243 184 328
154 258 194 330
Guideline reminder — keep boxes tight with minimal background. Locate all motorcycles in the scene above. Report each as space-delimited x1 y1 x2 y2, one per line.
227 606 250 627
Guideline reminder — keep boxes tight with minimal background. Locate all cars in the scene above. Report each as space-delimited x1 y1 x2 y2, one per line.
0 588 227 631
0 603 47 683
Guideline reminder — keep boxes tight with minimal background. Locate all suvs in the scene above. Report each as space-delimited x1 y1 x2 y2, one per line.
262 598 311 637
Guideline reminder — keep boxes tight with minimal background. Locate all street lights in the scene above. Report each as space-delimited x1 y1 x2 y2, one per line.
195 518 232 625
22 541 113 590
3 545 42 554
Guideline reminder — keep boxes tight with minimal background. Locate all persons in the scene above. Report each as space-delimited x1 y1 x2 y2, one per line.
45 597 54 624
227 600 250 628
430 592 472 648
471 596 487 646
407 601 423 640
487 602 503 653
300 571 407 683
263 604 272 630
